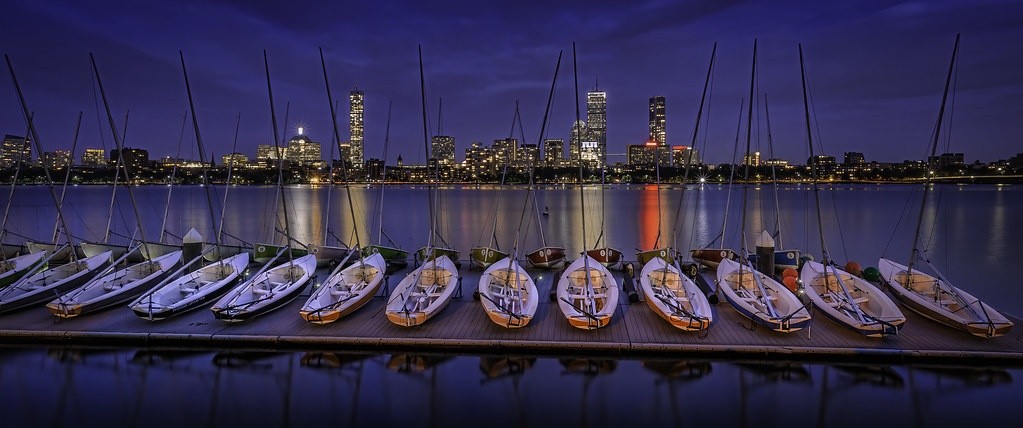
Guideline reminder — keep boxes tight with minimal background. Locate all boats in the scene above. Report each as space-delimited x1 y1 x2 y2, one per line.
1 59 114 315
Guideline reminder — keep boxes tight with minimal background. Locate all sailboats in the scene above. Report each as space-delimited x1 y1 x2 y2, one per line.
210 45 319 325
0 97 809 271
298 47 391 324
127 50 251 323
476 51 575 329
557 39 621 331
715 38 814 334
878 30 1013 339
791 43 908 338
640 46 719 334
384 43 459 328
41 47 184 319
